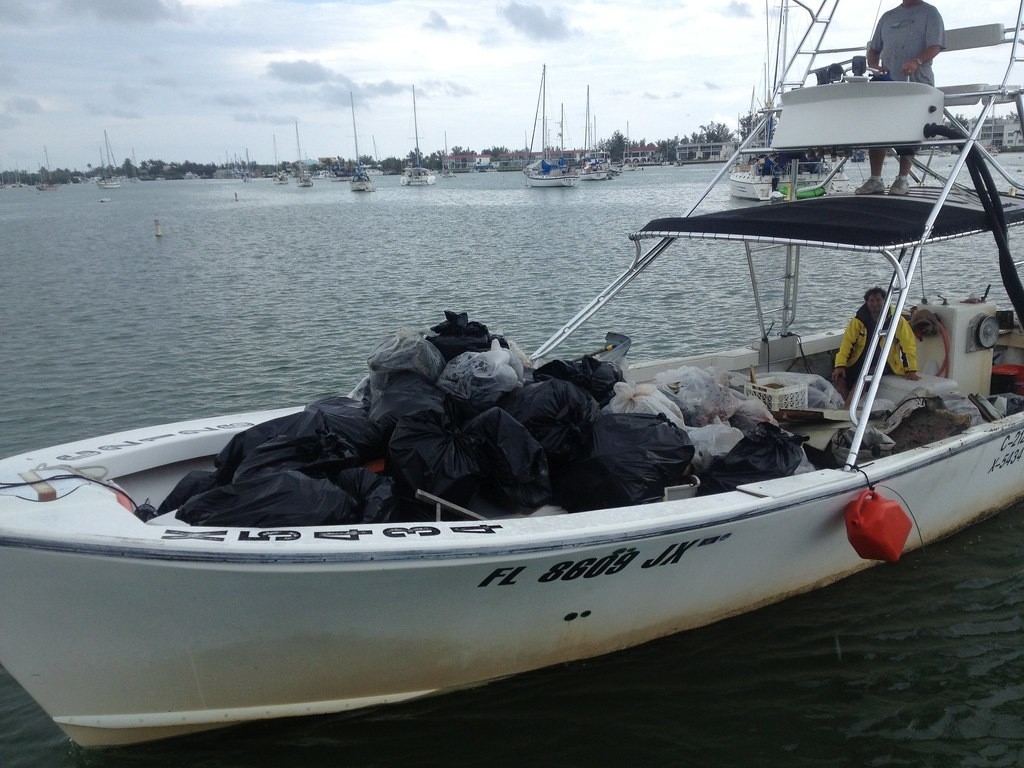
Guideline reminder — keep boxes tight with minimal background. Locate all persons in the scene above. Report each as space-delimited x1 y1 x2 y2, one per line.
854 0 947 197
831 285 922 410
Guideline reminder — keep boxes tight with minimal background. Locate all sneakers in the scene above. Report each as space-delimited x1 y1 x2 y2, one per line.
854 177 885 194
888 178 909 196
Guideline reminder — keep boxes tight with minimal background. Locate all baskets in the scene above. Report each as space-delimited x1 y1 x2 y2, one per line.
743 376 808 412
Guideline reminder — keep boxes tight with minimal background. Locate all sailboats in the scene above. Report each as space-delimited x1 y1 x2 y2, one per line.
271 134 289 185
732 0 856 200
345 90 377 192
400 85 438 186
523 65 642 186
96 130 127 189
294 122 315 187
223 148 253 182
1 163 59 191
440 130 457 178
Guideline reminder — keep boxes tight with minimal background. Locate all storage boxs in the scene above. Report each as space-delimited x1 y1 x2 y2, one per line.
743 377 809 411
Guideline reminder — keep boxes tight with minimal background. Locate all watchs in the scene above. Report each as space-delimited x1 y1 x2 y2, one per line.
913 57 922 65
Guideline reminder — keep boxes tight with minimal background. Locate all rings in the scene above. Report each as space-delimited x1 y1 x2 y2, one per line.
909 71 911 72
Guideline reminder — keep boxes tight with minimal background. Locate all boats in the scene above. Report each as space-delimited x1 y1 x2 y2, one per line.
155 177 166 180
313 164 384 178
0 1 1024 753
851 151 867 163
97 198 111 202
919 147 1001 158
468 167 499 174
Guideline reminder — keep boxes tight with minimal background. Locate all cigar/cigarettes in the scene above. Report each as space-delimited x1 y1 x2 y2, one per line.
832 373 834 374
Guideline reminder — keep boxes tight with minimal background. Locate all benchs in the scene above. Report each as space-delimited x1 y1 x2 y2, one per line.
874 373 959 401
